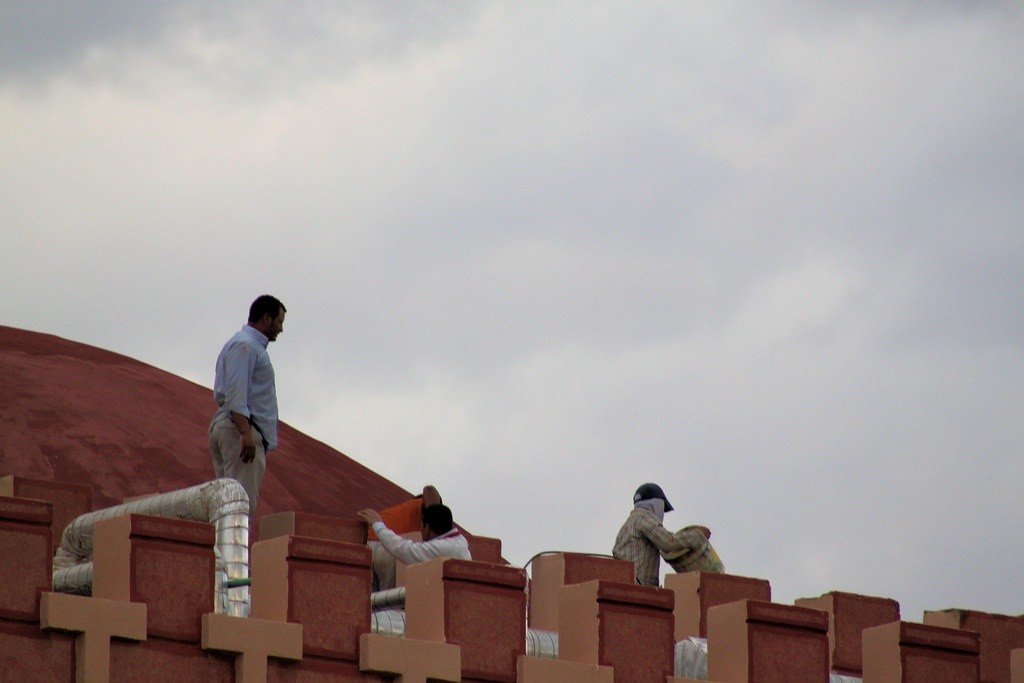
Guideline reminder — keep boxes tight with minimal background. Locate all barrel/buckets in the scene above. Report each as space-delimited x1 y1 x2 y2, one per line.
660 524 727 575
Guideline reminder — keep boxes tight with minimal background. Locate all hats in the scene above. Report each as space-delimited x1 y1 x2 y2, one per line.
634 483 674 512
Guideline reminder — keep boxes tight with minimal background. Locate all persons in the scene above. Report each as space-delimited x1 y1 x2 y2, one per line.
208 294 288 518
612 482 707 588
356 502 474 563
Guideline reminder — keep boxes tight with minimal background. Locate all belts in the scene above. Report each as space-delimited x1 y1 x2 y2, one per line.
249 417 270 454
